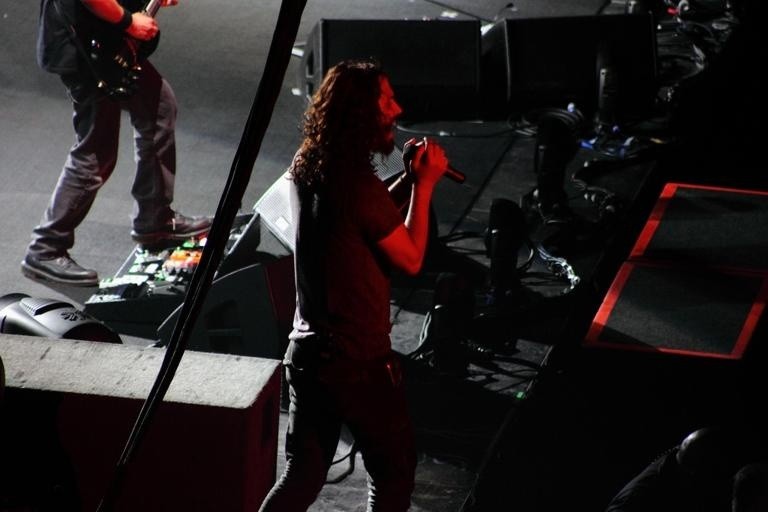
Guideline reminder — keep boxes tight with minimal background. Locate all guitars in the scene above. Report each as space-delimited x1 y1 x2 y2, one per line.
70 1 165 105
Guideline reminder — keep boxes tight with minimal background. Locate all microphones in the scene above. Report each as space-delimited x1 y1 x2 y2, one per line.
403 143 466 184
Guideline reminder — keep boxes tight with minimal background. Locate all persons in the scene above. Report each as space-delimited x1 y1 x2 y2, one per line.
256 57 450 512
20 0 216 288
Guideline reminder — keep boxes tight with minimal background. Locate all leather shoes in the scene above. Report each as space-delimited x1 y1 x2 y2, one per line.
131 211 211 242
21 250 98 286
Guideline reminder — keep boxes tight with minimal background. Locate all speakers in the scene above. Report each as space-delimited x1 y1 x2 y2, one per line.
155 258 293 364
300 17 485 123
482 13 658 121
0 293 122 344
